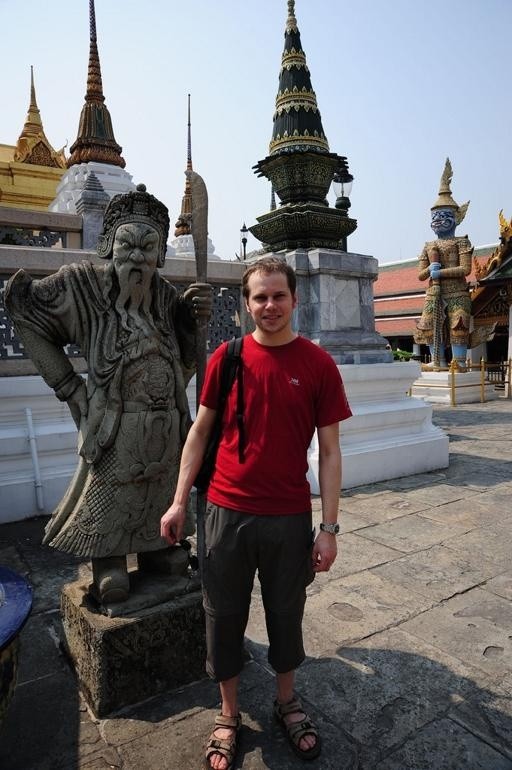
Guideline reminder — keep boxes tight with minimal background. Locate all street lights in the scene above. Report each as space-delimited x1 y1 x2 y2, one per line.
332 158 353 253
240 223 249 259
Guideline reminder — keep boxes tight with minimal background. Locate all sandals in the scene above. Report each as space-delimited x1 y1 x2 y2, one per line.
204 711 242 768
273 695 322 761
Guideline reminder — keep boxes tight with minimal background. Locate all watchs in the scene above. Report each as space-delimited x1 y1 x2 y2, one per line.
319 522 339 535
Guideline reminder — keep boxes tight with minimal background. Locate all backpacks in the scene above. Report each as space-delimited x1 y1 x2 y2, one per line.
193 336 246 494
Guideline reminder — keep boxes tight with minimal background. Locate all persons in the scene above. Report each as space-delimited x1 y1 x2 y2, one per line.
412 205 475 375
158 260 355 769
0 183 217 606
